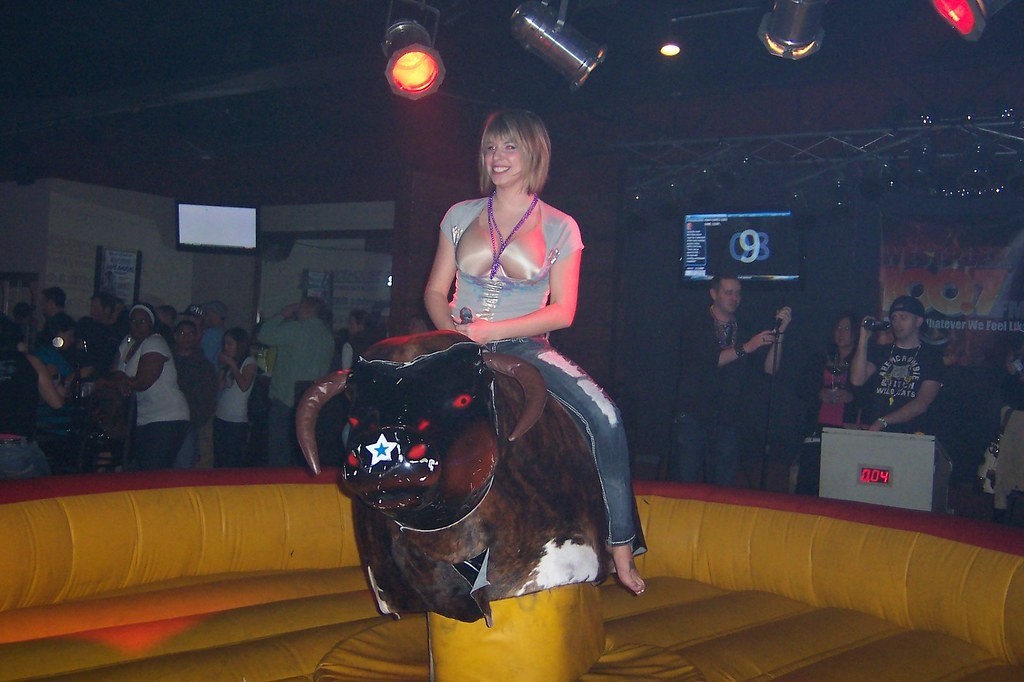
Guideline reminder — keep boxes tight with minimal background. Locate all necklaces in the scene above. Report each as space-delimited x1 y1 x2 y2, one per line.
888 340 921 406
831 353 842 389
488 188 537 280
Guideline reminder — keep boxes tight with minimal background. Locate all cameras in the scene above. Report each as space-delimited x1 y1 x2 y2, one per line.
863 319 892 332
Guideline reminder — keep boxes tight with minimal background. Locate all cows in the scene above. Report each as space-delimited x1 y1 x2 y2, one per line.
292 329 607 626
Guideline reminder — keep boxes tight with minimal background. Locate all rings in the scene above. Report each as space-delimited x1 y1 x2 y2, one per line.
763 338 765 342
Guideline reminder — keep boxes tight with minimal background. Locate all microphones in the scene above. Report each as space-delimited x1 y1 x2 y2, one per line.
772 307 793 334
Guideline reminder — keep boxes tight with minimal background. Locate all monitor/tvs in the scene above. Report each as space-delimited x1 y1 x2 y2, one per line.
173 199 263 257
680 211 804 285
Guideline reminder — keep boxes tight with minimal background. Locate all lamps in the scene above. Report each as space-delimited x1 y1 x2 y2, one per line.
756 0 824 60
928 0 1010 43
508 0 608 95
657 18 681 56
382 0 447 100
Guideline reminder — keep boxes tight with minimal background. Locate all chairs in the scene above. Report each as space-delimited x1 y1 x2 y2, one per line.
69 371 136 470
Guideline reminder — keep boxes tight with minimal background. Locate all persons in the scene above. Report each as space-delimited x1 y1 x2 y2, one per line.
423 106 648 594
795 294 1024 524
677 271 792 487
0 286 434 481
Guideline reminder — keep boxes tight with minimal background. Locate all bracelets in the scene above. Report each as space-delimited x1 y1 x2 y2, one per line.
878 416 887 428
773 341 782 343
780 331 784 336
735 343 746 358
